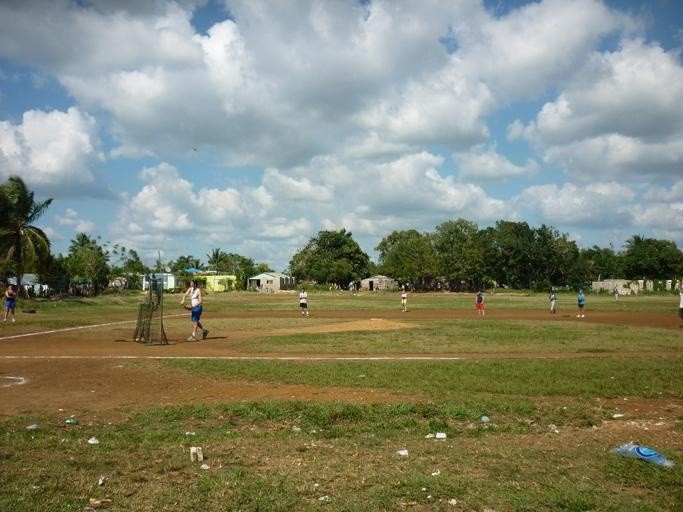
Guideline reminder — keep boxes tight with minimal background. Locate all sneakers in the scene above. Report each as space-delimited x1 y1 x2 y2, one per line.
575 315 585 318
186 329 209 342
3 319 15 322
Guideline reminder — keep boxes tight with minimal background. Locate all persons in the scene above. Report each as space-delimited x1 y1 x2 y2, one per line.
614 288 618 300
400 290 407 313
547 290 557 314
1 283 17 324
180 279 209 342
575 288 585 319
678 284 682 328
474 290 484 316
297 286 310 318
